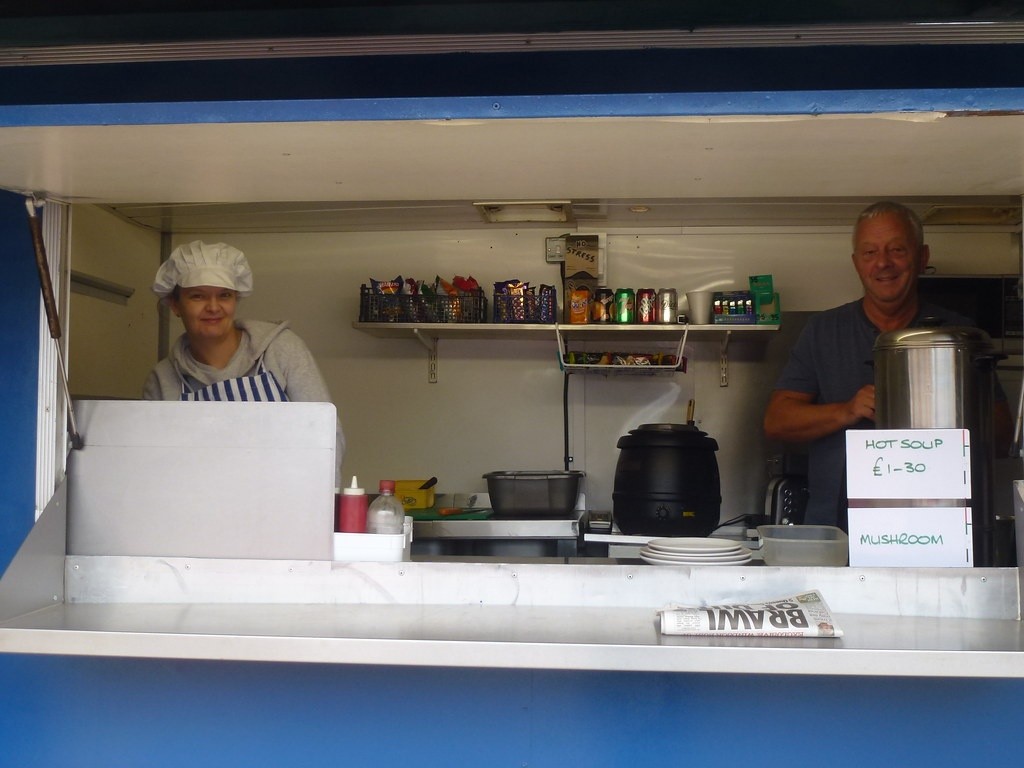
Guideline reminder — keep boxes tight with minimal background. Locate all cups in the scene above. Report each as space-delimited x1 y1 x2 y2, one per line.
686 290 714 325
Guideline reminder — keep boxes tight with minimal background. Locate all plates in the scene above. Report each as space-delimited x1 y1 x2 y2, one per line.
640 538 754 566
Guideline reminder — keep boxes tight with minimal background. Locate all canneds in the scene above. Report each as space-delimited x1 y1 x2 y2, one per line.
592 287 678 324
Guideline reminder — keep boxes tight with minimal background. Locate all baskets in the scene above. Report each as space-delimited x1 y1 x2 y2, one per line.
359 284 487 324
493 284 557 324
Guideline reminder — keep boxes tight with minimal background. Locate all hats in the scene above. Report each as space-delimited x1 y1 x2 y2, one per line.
152 240 254 298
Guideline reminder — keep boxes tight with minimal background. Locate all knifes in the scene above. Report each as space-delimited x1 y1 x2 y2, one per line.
437 507 484 515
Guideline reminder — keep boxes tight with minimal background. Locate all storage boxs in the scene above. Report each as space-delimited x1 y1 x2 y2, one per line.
334 515 413 562
494 289 557 324
359 283 488 324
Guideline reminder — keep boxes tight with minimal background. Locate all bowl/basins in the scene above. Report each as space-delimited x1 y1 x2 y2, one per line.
758 525 849 567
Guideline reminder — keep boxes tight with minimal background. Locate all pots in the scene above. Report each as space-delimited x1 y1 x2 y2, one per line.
482 469 585 517
863 315 1010 508
612 423 722 538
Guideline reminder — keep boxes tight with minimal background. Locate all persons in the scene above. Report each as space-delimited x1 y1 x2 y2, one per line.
146 240 345 487
765 201 1013 563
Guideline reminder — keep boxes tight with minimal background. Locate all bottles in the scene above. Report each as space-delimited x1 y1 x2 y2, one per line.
366 479 405 535
339 476 368 533
